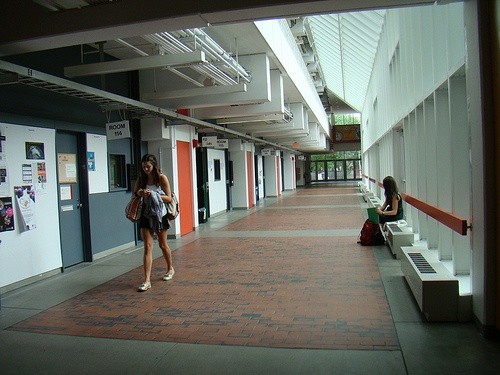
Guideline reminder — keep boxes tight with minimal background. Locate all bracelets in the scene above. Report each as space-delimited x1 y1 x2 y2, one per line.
382 211 384 215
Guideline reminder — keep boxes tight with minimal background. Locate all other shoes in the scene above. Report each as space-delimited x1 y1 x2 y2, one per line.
163 267 175 281
138 280 152 291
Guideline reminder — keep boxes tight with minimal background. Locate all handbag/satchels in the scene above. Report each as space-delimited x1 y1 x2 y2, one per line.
371 223 385 246
356 219 373 245
124 195 143 222
166 192 180 221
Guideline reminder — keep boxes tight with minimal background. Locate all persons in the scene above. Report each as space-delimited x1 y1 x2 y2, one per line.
356 176 403 243
132 154 175 291
0 200 14 231
